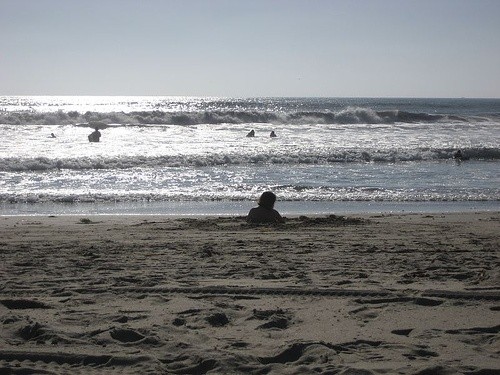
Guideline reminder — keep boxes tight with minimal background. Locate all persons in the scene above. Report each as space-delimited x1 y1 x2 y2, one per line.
88 126 101 142
49 133 56 138
246 130 254 137
246 192 285 225
270 131 276 137
